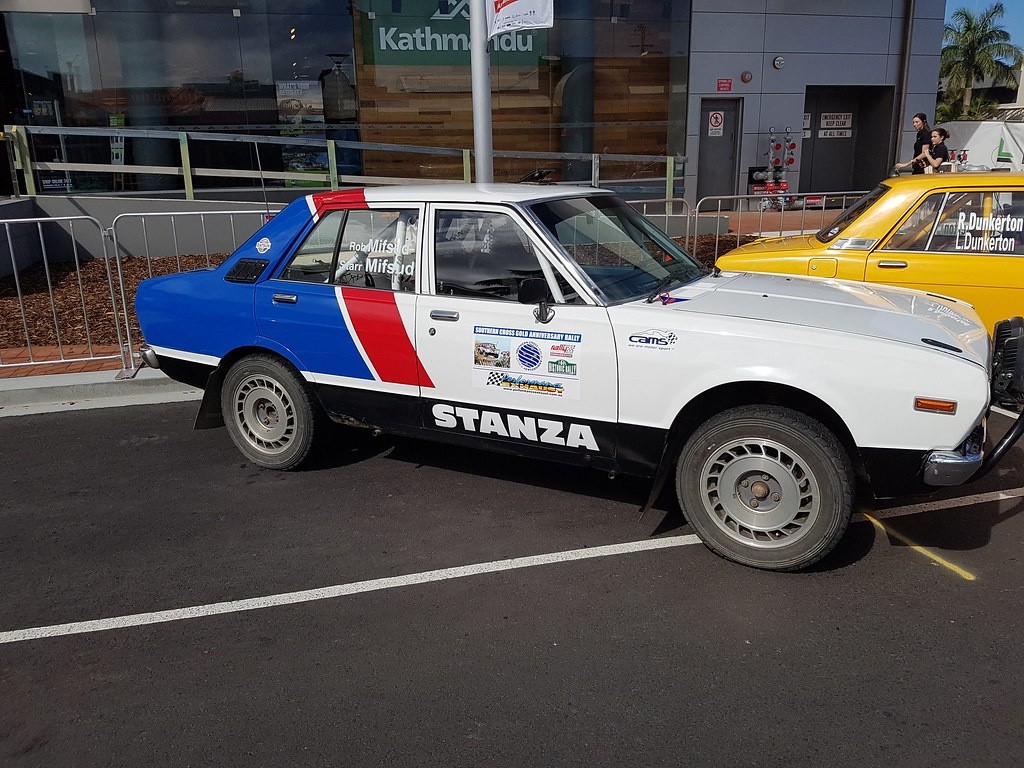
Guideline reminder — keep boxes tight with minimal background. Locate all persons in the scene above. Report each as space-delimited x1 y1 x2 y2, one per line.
894 113 934 175
919 128 950 173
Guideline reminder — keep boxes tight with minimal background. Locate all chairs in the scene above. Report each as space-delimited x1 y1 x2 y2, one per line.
942 206 982 253
488 226 551 273
434 237 501 295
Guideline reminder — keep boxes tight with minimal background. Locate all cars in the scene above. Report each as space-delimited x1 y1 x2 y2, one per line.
133 174 1024 572
715 166 1024 347
